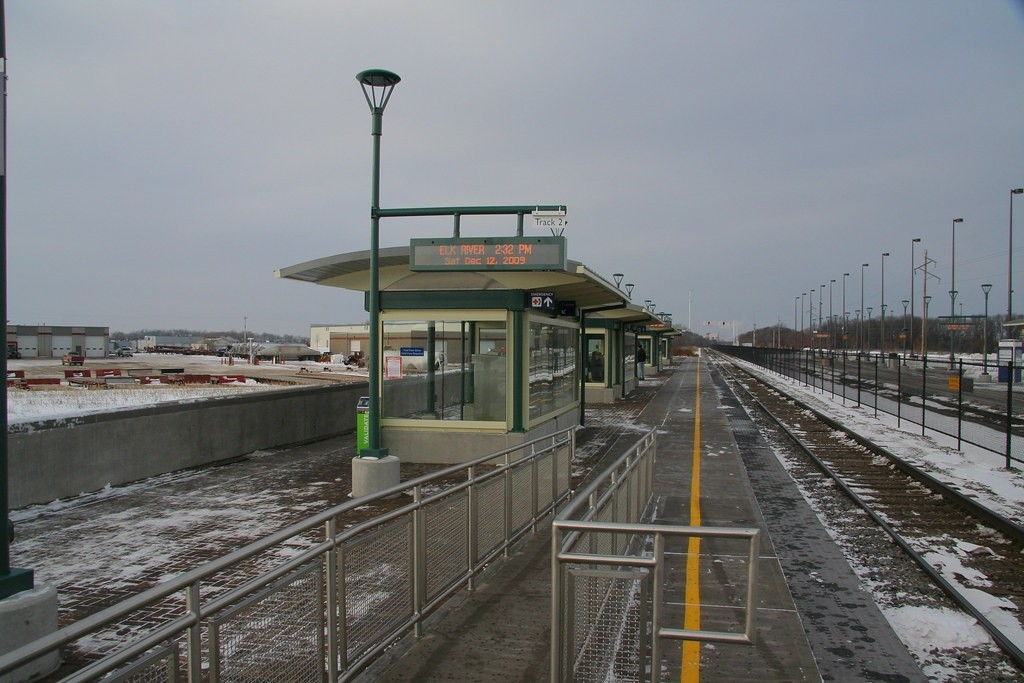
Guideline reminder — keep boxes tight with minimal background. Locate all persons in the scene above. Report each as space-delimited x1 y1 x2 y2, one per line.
636 345 647 381
828 351 833 365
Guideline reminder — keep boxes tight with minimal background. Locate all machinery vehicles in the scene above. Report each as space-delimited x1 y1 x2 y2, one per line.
341 350 368 366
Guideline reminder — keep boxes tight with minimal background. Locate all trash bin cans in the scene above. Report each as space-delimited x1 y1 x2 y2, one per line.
355 396 370 454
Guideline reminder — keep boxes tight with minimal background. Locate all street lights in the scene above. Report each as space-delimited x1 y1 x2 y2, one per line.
861 263 870 356
947 289 959 375
853 309 860 356
947 218 965 370
979 283 993 383
880 303 888 365
1006 188 1024 339
880 251 889 360
865 306 874 362
793 272 850 359
922 296 932 370
357 67 403 452
812 312 850 361
908 237 922 360
900 300 911 367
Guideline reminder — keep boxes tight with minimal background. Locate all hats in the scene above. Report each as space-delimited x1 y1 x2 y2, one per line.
638 345 642 349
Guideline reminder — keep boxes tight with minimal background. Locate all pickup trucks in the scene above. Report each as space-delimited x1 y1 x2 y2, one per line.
62 351 85 366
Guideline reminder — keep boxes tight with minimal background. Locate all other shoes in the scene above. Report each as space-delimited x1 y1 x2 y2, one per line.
639 378 644 380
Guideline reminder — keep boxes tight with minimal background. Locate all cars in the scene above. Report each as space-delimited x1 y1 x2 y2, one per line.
115 347 134 357
216 347 227 357
